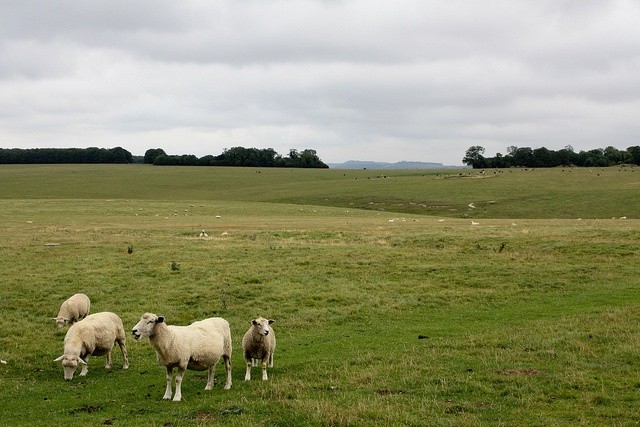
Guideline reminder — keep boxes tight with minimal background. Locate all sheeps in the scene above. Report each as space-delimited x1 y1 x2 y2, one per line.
51 293 92 328
53 311 129 382
241 315 277 381
131 311 234 403
